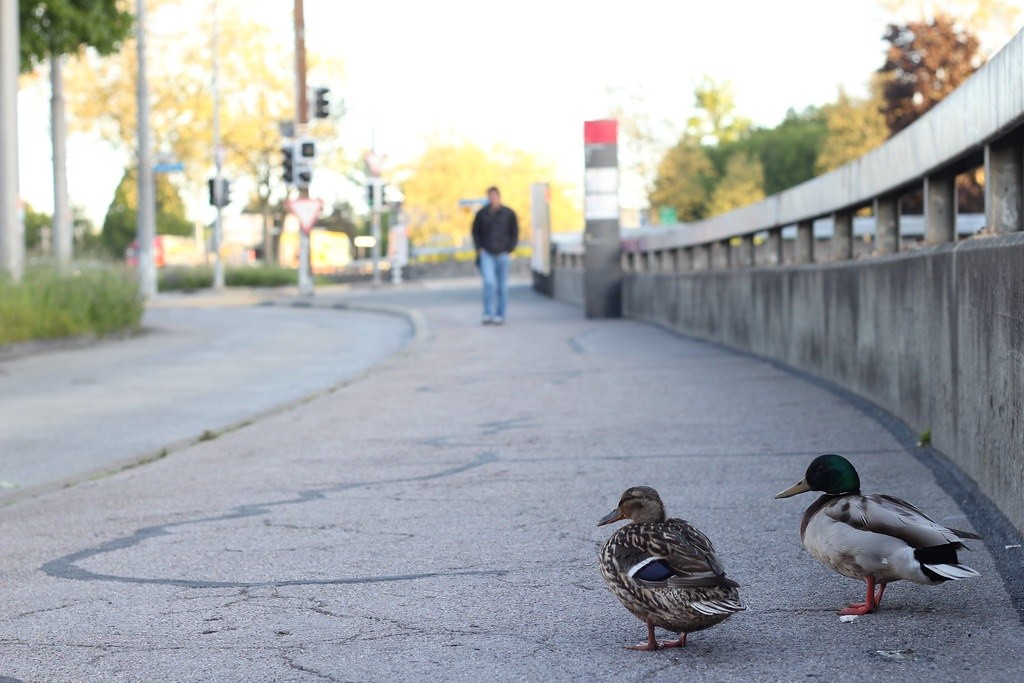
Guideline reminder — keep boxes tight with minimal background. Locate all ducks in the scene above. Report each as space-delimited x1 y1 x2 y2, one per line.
597 486 748 651
774 454 986 615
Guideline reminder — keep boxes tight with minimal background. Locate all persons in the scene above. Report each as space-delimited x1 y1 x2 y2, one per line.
472 188 518 325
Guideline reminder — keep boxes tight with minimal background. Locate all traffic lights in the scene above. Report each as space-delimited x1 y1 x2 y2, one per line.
280 141 295 184
295 136 317 189
313 88 330 118
219 178 232 206
207 179 218 207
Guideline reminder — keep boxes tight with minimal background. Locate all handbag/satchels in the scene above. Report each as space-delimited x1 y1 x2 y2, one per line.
474 255 480 266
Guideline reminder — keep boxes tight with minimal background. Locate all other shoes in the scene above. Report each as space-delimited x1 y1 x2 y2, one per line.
482 315 504 325
